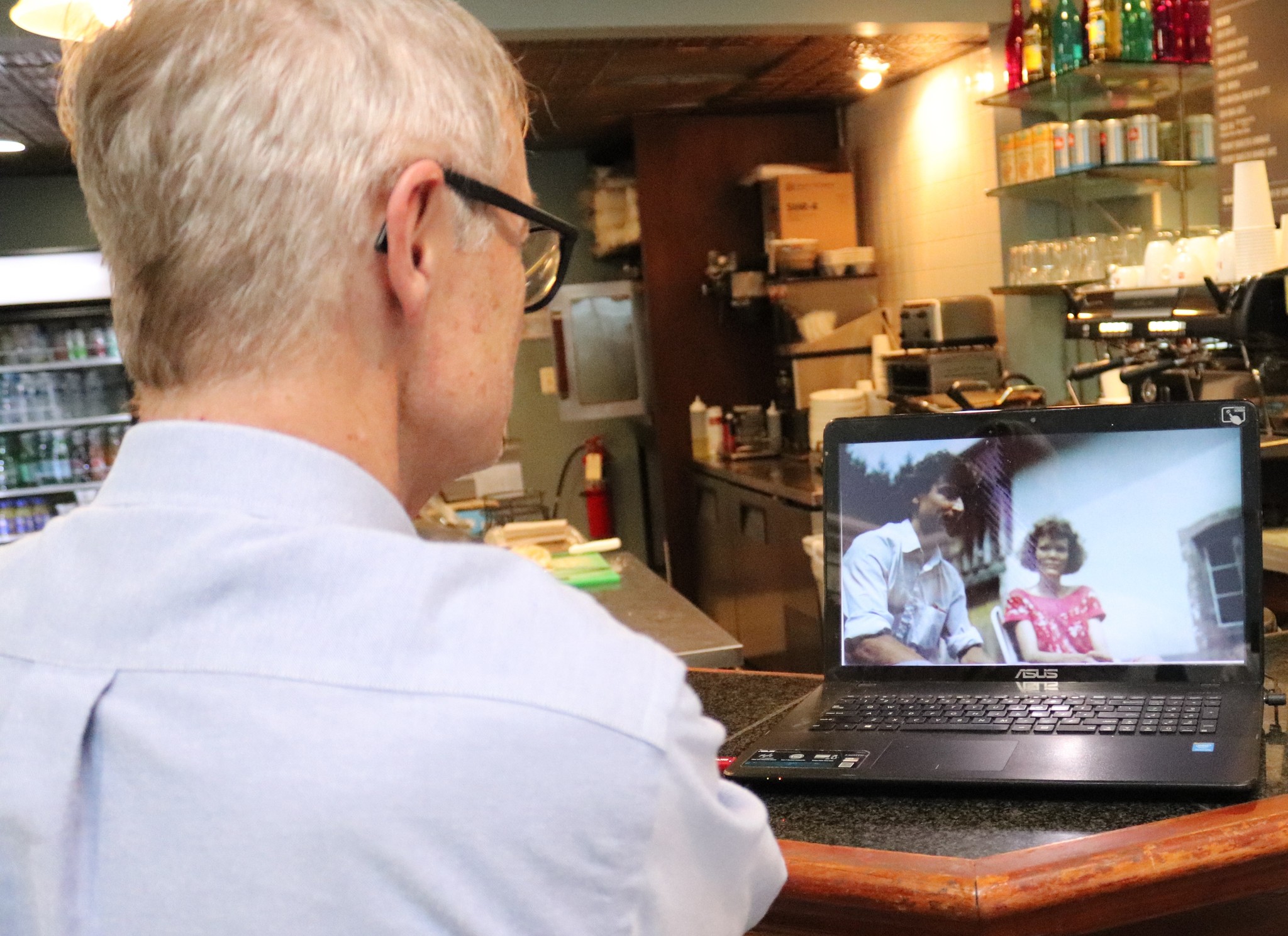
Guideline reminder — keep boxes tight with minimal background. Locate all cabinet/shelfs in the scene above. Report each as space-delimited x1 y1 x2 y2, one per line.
982 61 1215 297
713 278 879 423
693 475 829 673
0 250 133 544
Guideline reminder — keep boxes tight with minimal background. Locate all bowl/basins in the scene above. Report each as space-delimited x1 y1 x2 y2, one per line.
796 310 837 340
820 248 849 276
849 246 875 275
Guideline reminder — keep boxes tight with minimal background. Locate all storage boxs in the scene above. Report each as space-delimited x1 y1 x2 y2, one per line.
444 461 523 505
761 172 857 269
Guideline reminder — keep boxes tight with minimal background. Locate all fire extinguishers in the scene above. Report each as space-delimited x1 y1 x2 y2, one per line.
582 435 615 539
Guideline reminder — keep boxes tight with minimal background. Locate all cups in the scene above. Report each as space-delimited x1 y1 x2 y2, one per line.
856 378 872 392
1009 224 1222 286
872 334 892 390
1109 160 1288 289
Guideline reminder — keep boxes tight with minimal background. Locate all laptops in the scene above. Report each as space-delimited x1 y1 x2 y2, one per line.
720 398 1266 797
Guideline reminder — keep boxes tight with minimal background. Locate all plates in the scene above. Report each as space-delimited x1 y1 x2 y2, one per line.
808 388 868 451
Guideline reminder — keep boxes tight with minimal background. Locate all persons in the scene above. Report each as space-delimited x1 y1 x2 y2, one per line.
840 454 1000 666
1002 519 1113 663
1 0 791 935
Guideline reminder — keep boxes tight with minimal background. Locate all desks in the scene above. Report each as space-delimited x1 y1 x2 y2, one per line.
679 651 1288 936
580 549 746 670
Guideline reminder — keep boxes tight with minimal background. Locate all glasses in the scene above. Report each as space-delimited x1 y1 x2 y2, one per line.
374 170 582 316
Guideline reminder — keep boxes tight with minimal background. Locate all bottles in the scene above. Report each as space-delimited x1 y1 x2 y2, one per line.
766 400 782 450
1004 0 1212 91
0 324 120 366
705 405 724 465
0 365 134 425
723 413 736 454
689 395 707 464
0 496 57 536
0 424 132 490
